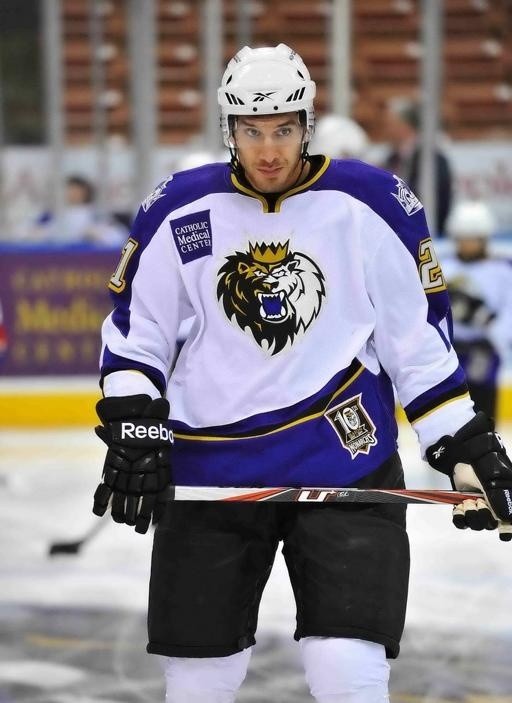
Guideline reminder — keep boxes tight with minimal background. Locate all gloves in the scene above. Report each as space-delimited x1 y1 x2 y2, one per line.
426 411 512 542
92 395 172 533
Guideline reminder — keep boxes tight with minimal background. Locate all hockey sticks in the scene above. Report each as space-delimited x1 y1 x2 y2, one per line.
48 506 113 555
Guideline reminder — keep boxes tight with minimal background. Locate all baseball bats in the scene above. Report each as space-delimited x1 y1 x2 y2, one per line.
170 484 511 541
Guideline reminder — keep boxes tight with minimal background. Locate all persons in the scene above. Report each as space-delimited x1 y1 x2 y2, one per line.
424 208 512 428
27 175 99 237
377 98 452 238
91 42 511 702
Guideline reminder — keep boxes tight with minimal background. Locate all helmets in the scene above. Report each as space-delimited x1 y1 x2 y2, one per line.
219 44 315 145
